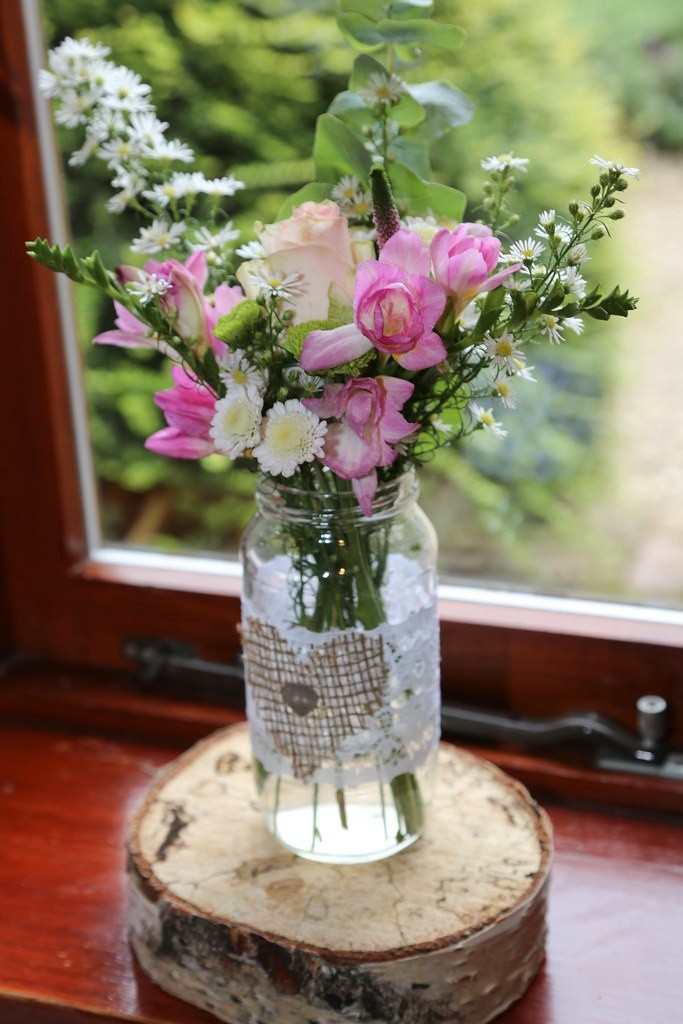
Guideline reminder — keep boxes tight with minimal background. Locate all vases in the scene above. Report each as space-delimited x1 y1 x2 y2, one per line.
238 458 443 865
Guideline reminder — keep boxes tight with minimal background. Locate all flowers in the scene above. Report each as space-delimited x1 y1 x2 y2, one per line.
31 37 644 512
